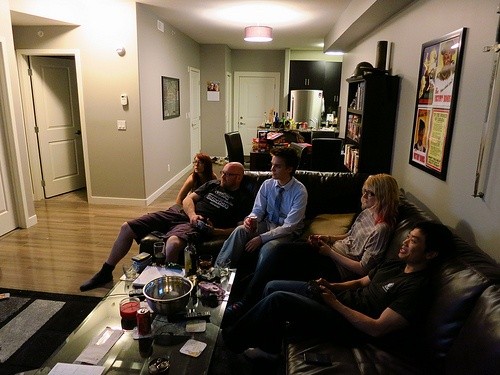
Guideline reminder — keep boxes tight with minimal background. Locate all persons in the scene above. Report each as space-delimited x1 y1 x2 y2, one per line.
176 153 217 204
221 221 454 365
79 162 251 292
225 174 399 320
214 146 308 268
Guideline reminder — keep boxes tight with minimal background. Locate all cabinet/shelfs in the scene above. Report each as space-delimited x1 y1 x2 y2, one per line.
342 74 400 173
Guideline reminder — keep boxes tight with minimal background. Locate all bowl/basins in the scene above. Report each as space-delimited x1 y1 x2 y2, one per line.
143 275 194 315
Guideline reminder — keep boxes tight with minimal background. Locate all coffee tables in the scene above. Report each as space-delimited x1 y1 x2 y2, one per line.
32 263 237 375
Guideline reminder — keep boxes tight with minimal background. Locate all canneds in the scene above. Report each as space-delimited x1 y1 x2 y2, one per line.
137 308 151 335
249 216 258 233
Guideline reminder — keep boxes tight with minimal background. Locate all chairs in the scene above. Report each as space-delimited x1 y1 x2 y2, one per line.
225 130 344 174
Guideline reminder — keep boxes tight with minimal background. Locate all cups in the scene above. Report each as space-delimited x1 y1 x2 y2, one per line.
119 297 141 320
148 353 170 375
121 260 138 279
197 254 213 273
153 242 166 265
217 257 232 279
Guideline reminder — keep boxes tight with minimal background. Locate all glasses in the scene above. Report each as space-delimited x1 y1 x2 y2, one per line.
364 189 375 196
220 171 241 176
193 161 203 164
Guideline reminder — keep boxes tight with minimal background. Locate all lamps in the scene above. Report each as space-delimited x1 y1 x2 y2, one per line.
243 25 273 43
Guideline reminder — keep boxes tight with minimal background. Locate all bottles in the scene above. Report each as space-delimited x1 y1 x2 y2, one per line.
263 110 290 129
184 242 197 276
136 308 150 331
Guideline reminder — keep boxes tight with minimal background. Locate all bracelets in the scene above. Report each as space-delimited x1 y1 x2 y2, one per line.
328 235 331 244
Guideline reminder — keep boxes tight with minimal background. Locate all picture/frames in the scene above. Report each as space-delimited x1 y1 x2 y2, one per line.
408 27 467 182
162 75 180 120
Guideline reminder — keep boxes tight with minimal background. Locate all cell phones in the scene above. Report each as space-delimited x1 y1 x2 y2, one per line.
304 352 332 367
132 252 150 262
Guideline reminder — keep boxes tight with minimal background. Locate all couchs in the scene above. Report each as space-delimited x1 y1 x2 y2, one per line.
283 195 500 375
138 170 367 263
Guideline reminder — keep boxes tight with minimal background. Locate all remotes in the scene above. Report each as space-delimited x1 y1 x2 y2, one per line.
168 311 210 323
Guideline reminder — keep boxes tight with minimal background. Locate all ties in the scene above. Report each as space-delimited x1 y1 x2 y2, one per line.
272 188 285 223
